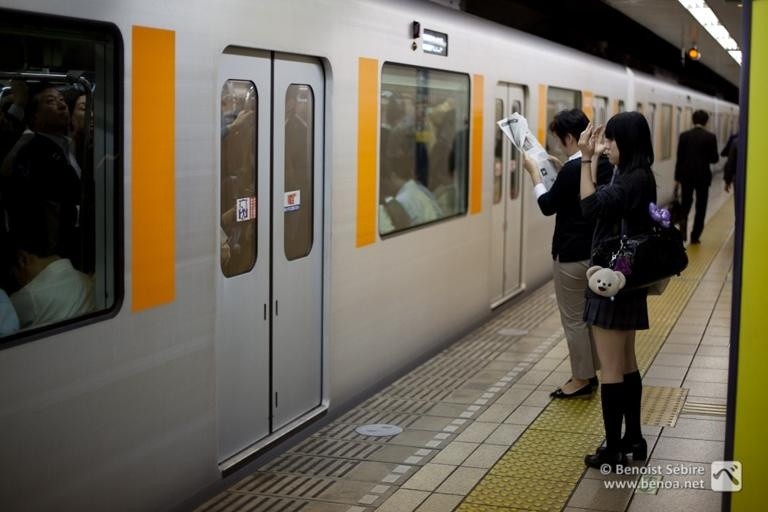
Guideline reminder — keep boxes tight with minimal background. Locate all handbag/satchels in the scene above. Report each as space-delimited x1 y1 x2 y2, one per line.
667 184 687 241
590 233 688 287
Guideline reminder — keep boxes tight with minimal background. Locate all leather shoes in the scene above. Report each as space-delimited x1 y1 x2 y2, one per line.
550 373 599 400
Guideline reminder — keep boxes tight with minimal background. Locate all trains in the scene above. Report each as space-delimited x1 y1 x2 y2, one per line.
0 1 739 512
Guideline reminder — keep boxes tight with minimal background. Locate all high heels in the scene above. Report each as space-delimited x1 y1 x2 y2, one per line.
584 447 627 472
597 438 647 460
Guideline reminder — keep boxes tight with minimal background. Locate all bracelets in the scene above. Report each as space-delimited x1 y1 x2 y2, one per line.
580 160 593 164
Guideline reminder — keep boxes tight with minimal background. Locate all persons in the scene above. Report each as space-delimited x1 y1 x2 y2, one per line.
0 66 95 341
577 111 657 474
220 79 314 278
721 133 738 156
522 109 613 399
585 265 627 298
379 88 470 235
674 110 719 245
723 138 736 196
